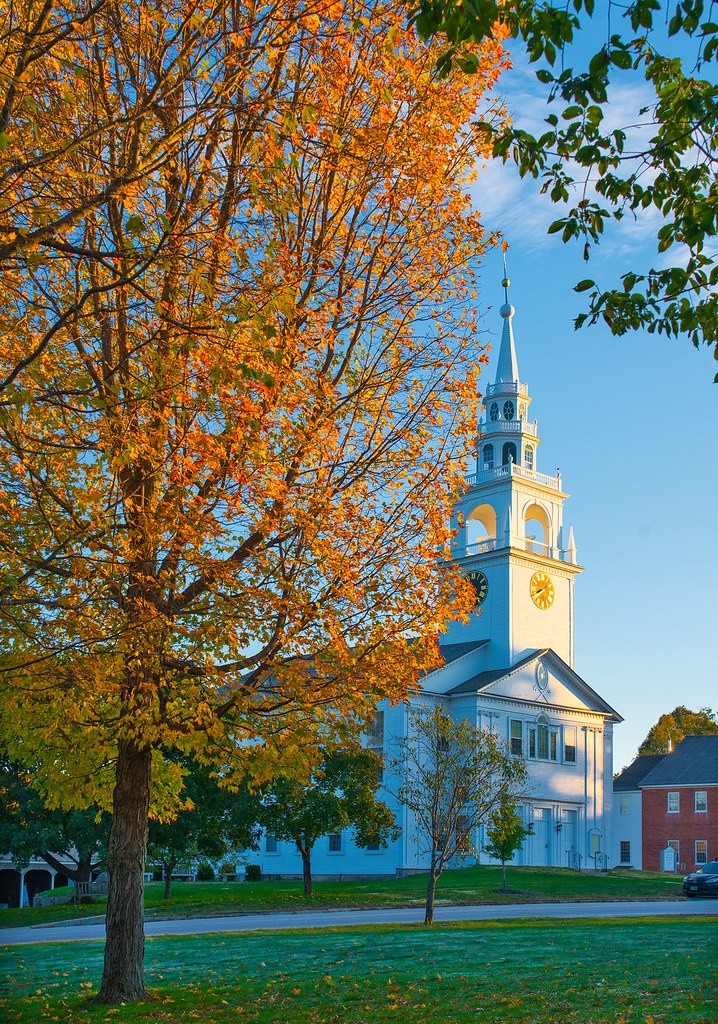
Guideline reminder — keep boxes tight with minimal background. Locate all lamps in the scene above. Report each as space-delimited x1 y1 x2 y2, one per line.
529 822 535 829
557 822 562 834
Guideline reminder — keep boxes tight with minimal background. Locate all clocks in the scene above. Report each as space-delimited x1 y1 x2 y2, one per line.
457 569 487 609
530 571 555 610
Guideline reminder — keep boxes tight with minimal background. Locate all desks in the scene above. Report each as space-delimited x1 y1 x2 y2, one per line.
220 872 250 883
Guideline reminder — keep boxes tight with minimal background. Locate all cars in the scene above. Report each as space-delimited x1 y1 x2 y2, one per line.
682 858 718 898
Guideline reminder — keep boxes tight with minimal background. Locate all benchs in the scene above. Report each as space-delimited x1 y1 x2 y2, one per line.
163 868 197 882
72 881 109 903
